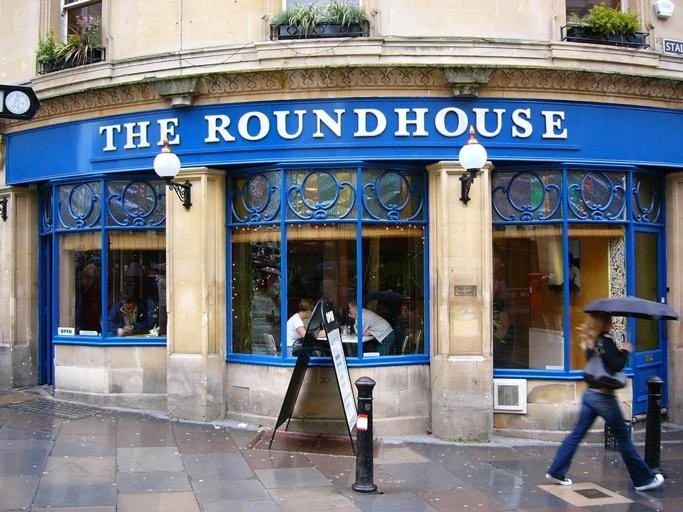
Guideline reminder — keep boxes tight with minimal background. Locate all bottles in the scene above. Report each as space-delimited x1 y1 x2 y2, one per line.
342 324 352 336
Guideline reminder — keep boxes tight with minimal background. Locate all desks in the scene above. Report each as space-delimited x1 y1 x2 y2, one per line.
123 333 166 337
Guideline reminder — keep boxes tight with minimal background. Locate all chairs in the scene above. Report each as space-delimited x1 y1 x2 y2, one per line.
262 332 281 356
401 335 410 355
404 326 423 354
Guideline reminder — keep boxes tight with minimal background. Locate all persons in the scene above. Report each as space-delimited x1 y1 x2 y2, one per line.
287 297 327 357
108 288 155 335
546 313 664 491
348 300 396 355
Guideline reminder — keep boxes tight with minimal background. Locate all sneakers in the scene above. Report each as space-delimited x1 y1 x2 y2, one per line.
545 474 573 486
634 474 665 491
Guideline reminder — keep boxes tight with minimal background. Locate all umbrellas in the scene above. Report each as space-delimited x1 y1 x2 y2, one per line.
582 295 679 321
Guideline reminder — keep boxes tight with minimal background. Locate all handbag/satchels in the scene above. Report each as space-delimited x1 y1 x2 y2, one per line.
583 352 626 389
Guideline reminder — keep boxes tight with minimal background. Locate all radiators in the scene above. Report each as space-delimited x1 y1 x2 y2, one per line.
528 327 564 369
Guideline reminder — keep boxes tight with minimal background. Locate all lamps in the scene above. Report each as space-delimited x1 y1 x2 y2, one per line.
153 138 194 210
456 123 487 204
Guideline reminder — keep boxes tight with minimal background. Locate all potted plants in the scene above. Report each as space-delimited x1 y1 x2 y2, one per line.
560 0 649 49
269 0 371 41
37 31 106 74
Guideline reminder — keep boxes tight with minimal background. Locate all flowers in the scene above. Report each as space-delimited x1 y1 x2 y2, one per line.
75 13 103 46
